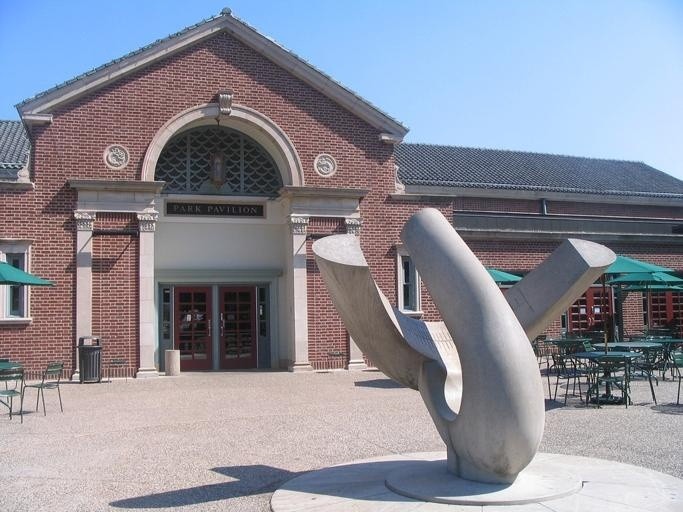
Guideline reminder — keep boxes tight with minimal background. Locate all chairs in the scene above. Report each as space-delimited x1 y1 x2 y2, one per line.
0 366 24 424
532 336 683 409
19 362 65 416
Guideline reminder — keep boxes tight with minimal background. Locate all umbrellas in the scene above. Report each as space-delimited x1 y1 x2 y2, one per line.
604 254 677 353
485 267 524 285
604 271 683 337
0 259 57 289
620 284 683 329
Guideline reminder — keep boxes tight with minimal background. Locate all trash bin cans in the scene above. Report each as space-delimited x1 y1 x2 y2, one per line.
76 336 103 384
